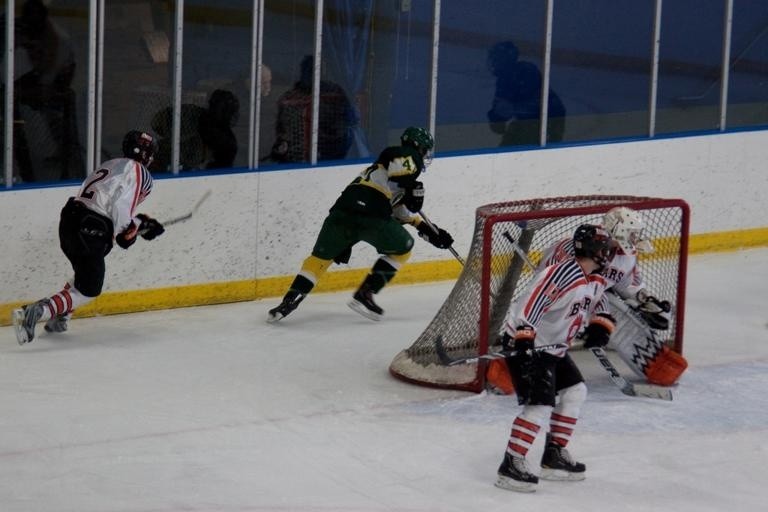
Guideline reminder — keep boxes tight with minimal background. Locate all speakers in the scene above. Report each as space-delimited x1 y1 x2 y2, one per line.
21 298 50 342
268 291 303 317
353 274 382 315
540 432 585 472
497 452 538 484
43 311 67 332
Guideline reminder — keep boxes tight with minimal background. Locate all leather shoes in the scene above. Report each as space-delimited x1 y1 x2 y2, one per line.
135 213 164 240
417 221 453 249
579 313 616 349
115 219 137 249
514 325 535 363
403 181 424 214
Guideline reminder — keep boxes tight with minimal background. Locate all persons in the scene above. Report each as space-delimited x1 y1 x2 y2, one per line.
534 207 687 386
20 128 167 343
222 64 274 170
486 39 567 151
273 56 356 162
150 90 240 173
269 125 453 319
498 224 627 484
10 42 86 185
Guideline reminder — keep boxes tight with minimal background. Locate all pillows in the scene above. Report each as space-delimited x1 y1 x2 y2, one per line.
122 129 158 167
603 206 642 256
572 224 611 266
400 126 433 171
